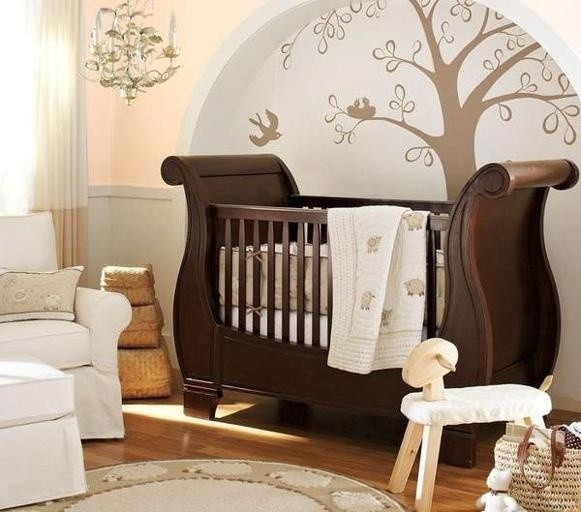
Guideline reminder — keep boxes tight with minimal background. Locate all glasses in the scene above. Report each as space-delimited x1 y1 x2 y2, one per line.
77 0 184 106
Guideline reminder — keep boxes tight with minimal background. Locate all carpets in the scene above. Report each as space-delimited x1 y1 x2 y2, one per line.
0 459 404 510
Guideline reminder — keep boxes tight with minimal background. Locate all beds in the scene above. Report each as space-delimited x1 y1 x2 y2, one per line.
160 155 579 468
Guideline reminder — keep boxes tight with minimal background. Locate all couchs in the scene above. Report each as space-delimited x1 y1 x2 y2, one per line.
2 352 87 509
0 209 133 438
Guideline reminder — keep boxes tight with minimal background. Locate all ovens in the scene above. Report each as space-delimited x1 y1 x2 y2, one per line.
160 155 579 468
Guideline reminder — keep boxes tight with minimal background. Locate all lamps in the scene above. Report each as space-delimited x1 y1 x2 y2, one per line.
77 0 184 106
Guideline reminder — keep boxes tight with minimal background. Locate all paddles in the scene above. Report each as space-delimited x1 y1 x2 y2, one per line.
0 459 404 510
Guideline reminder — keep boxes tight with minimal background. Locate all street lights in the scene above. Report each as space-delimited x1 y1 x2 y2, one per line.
494 422 581 511
